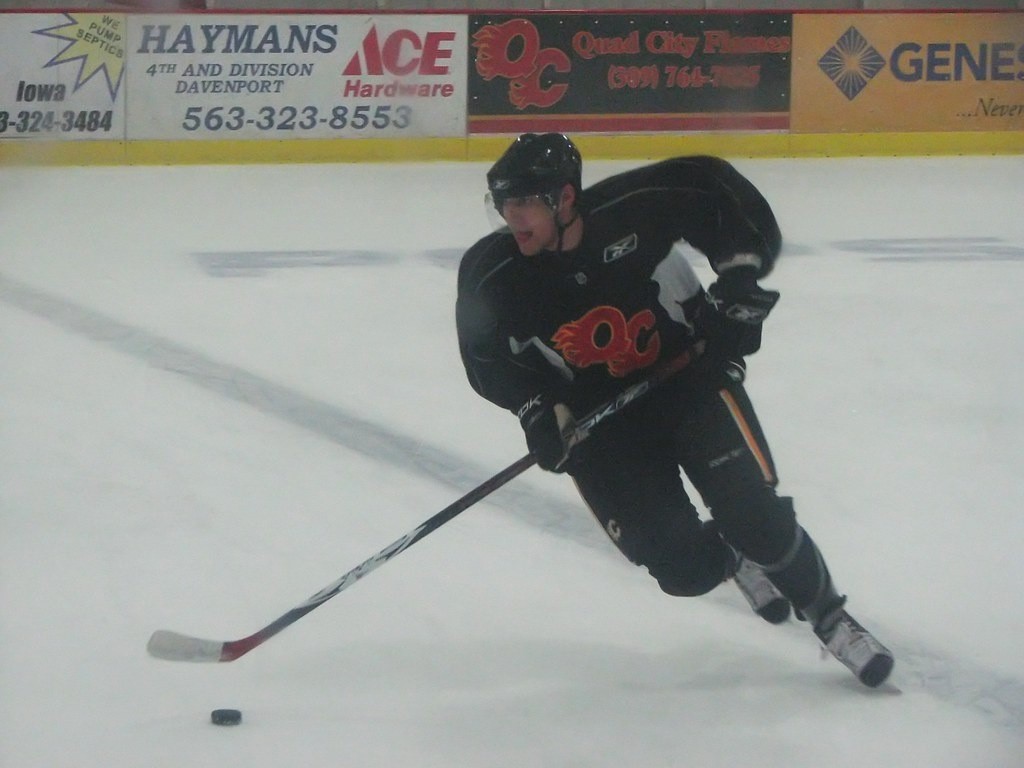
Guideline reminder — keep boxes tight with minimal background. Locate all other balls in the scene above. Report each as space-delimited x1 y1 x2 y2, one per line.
211 708 243 727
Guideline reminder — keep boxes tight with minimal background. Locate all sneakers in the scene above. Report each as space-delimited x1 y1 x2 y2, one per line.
704 520 793 626
813 595 902 692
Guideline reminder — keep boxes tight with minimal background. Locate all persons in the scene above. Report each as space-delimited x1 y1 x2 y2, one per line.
455 132 896 692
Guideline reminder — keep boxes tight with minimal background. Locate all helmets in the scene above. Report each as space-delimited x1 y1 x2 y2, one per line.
484 133 581 215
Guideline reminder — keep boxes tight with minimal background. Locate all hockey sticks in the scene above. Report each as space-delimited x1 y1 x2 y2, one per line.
145 341 702 664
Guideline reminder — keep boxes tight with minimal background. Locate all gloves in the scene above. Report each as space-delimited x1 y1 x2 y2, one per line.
516 391 575 473
693 283 780 357
